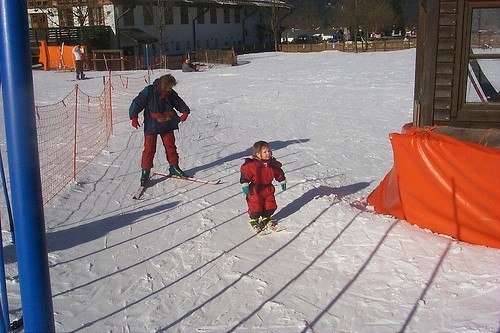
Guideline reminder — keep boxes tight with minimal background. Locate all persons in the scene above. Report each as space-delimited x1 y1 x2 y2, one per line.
182 59 199 72
129 74 190 187
241 140 287 232
71 45 87 80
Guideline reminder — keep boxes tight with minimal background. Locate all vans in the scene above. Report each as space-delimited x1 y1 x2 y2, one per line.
292 34 323 44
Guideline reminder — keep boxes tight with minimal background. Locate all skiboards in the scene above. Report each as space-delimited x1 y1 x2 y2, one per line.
246 215 283 235
132 171 220 200
66 77 94 82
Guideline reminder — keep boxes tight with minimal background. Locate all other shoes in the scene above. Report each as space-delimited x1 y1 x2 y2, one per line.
76 74 81 80
81 74 85 79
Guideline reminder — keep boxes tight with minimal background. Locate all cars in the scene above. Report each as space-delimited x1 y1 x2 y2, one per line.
356 31 375 41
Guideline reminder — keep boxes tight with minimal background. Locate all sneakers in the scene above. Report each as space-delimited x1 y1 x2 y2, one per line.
248 218 260 230
260 218 275 227
169 165 189 178
141 169 151 185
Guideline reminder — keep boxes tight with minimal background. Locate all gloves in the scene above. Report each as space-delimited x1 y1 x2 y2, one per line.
280 180 287 191
241 182 252 195
132 117 140 130
179 112 189 121
